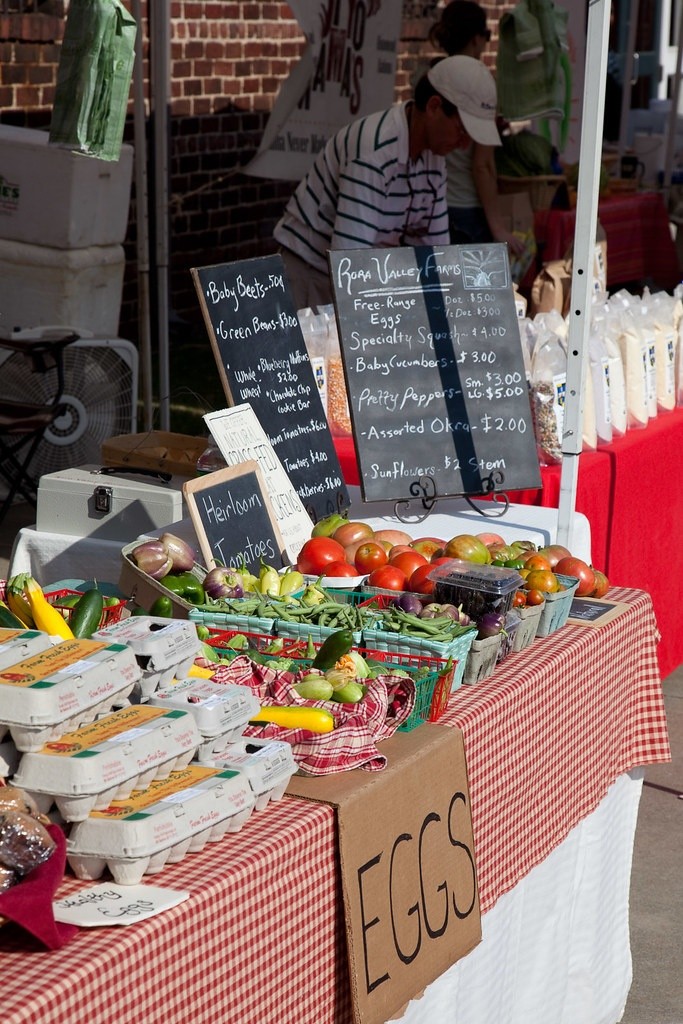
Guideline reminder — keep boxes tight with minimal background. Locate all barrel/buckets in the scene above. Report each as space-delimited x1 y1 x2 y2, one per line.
633 131 668 185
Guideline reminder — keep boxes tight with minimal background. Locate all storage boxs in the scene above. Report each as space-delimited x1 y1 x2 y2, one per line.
119 539 208 619
278 565 582 686
0 123 133 341
36 429 228 541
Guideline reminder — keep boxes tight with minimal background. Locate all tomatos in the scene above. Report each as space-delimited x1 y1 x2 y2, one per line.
295 517 607 608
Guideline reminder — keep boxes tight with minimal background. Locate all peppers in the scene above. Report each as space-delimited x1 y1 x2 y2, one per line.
390 592 512 639
126 532 245 604
209 553 326 601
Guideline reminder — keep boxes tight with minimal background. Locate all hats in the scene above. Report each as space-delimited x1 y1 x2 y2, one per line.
428 55 506 146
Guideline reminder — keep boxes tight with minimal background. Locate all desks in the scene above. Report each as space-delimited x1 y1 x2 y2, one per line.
7 524 188 619
332 408 683 680
0 586 672 1022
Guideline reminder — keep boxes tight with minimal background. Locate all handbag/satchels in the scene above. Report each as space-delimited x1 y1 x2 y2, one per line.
101 388 212 477
495 191 537 284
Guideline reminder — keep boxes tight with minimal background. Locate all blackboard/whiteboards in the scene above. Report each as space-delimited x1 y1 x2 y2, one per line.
193 255 352 527
185 463 288 586
327 242 544 502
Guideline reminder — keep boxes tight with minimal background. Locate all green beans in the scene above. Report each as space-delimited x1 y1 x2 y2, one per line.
190 584 478 643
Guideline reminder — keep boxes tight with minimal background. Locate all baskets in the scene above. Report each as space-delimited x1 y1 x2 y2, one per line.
498 175 558 211
196 573 582 732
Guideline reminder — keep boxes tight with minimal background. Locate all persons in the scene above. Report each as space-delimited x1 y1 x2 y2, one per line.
273 56 503 316
413 0 529 258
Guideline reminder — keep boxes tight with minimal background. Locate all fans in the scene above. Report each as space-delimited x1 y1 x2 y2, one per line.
1 337 139 497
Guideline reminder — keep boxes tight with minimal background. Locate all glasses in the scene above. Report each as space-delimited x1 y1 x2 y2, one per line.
475 27 494 42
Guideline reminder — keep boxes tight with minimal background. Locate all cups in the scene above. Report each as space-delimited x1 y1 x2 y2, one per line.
620 155 645 180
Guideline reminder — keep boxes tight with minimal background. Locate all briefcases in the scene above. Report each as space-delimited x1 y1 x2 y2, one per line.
36 461 196 541
